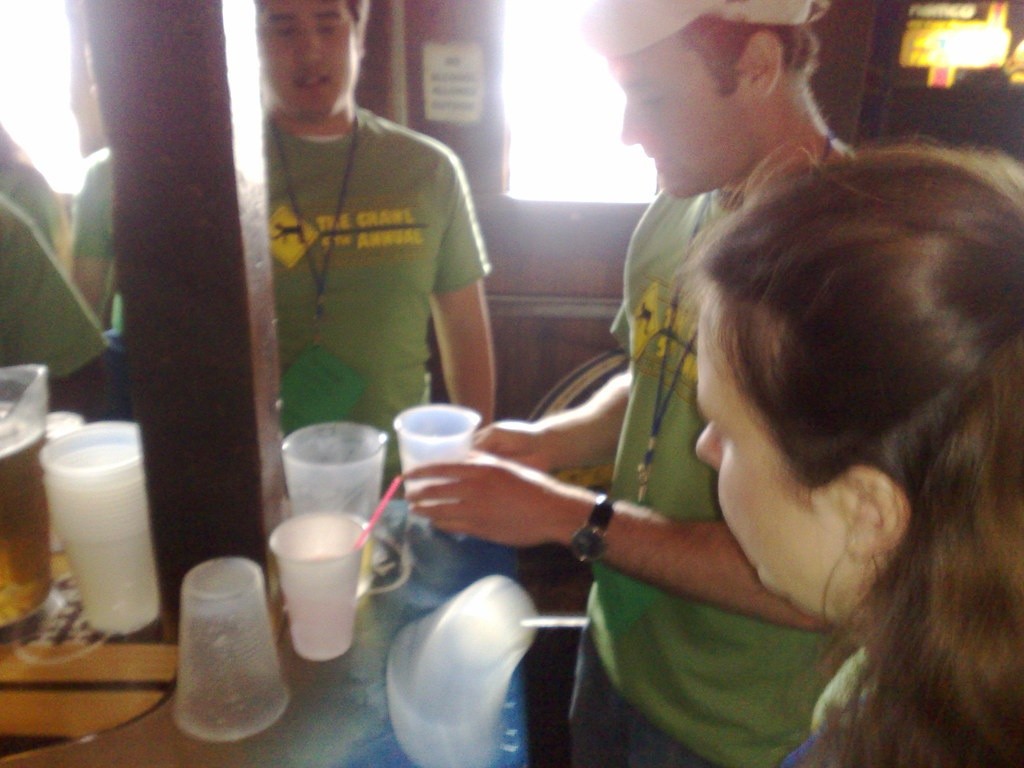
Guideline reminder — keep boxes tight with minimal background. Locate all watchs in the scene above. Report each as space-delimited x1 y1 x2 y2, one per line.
561 493 615 566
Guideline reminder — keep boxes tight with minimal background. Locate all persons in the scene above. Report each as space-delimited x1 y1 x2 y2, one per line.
404 1 875 768
253 0 496 497
694 140 1024 768
1 34 128 431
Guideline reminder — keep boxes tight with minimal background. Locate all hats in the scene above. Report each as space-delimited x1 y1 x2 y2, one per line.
573 0 832 61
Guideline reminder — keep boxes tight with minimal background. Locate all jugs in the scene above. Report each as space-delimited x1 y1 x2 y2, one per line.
0 363 48 643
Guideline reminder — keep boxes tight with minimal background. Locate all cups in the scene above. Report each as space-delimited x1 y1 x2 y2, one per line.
386 575 540 768
270 513 369 662
281 422 389 599
175 558 292 742
393 403 484 510
39 411 161 634
